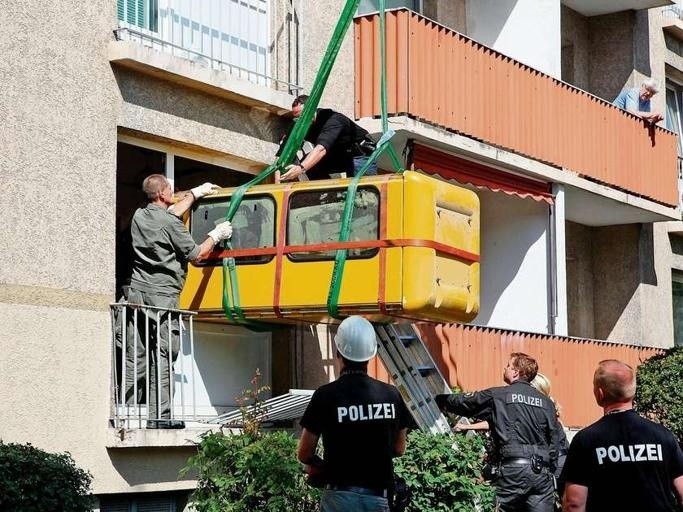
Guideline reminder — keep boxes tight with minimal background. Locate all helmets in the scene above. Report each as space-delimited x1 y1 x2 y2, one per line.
334 316 378 362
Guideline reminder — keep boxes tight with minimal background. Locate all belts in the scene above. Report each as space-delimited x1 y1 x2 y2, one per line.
504 456 550 467
325 483 384 497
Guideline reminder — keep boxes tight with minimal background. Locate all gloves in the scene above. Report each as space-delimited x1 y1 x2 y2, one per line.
207 221 233 246
191 181 222 202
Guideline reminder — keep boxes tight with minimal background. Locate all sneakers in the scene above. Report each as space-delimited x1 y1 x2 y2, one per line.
147 420 185 429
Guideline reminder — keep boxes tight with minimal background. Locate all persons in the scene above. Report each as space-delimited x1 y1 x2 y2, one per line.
453 373 570 478
434 352 560 512
557 360 683 512
613 78 664 124
274 95 378 184
298 314 418 512
114 174 233 429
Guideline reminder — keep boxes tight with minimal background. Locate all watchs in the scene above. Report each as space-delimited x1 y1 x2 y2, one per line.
298 164 306 174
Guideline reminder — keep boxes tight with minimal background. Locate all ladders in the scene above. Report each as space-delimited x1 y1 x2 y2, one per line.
371 321 500 512
